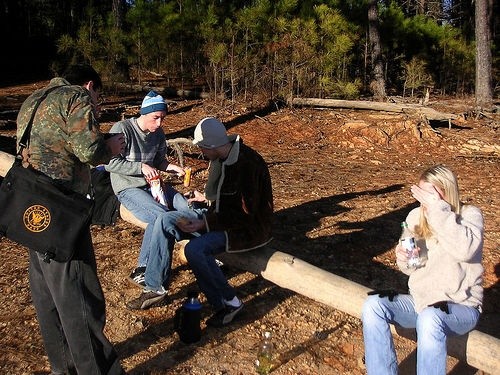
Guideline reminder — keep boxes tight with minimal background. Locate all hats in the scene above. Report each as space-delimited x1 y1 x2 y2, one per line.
140 90 168 114
193 117 227 149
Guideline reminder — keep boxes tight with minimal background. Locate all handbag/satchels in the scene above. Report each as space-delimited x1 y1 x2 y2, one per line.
0 153 95 263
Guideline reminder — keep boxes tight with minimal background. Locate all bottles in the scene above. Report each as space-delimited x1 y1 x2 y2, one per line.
400 221 421 269
174 296 203 344
254 330 274 375
188 191 195 199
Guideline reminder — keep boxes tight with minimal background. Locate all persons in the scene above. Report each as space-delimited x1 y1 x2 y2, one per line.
123 117 275 328
104 90 189 290
362 164 484 375
17 64 128 375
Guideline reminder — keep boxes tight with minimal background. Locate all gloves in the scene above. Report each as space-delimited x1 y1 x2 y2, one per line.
368 287 398 302
428 301 449 314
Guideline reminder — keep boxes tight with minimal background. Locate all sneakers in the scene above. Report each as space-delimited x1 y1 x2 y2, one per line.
127 287 170 308
127 268 146 285
205 300 244 328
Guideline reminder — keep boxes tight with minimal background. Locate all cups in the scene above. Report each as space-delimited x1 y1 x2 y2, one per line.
104 132 125 153
